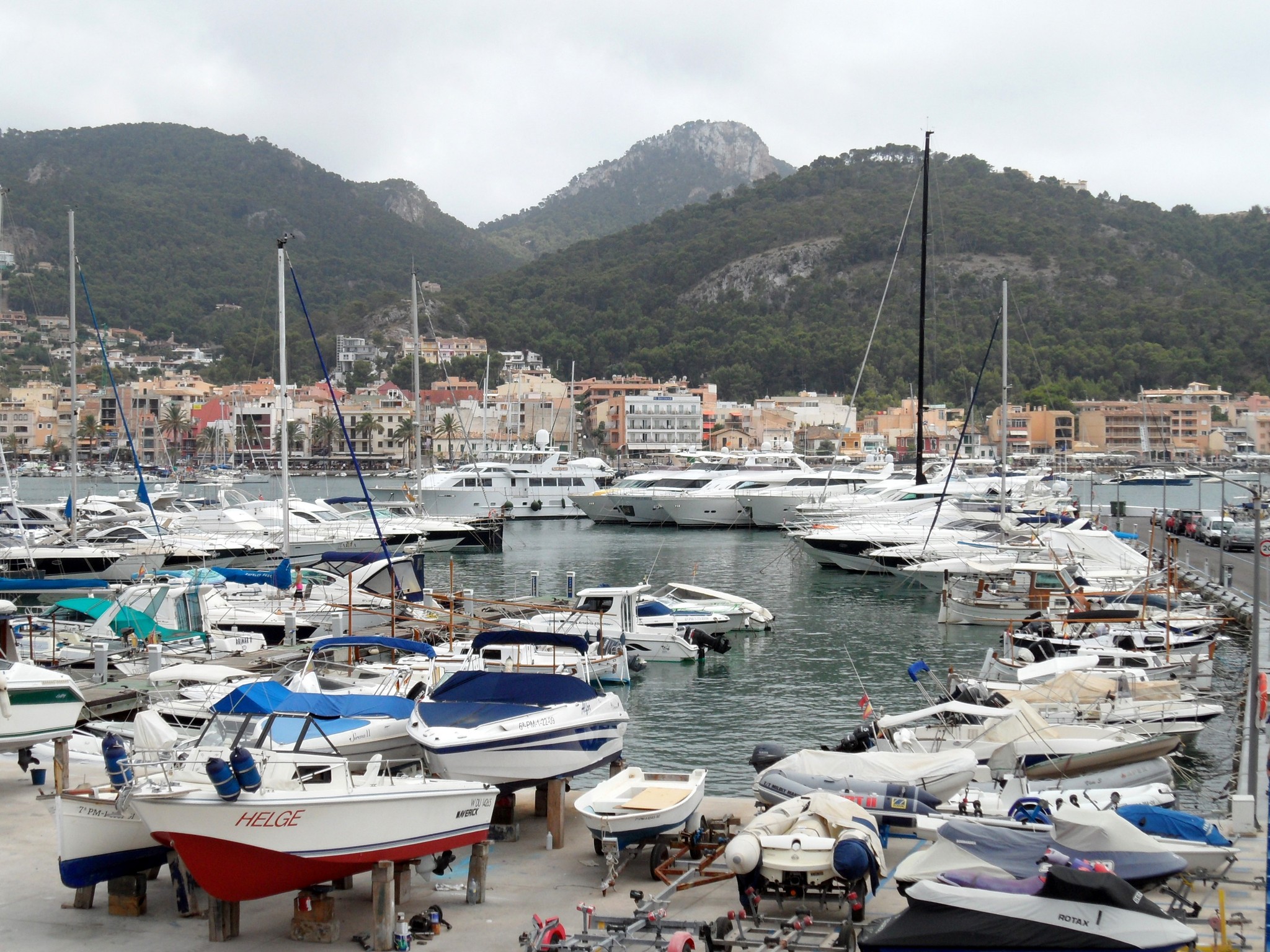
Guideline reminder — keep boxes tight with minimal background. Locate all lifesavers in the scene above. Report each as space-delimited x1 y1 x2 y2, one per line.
488 509 497 519
127 632 138 646
1259 672 1268 719
1092 490 1095 500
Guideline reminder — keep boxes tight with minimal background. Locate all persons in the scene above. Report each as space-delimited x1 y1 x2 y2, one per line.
288 565 306 611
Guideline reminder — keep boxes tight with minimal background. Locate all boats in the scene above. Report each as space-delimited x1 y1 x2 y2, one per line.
574 765 708 847
120 747 502 905
720 791 881 889
1 139 1269 951
402 630 631 795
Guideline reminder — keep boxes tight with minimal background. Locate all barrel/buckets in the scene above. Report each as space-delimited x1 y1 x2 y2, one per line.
394 931 412 952
30 769 46 785
430 912 439 923
431 922 441 935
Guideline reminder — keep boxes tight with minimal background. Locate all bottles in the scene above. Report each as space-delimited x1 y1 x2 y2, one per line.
546 831 553 850
468 878 476 906
